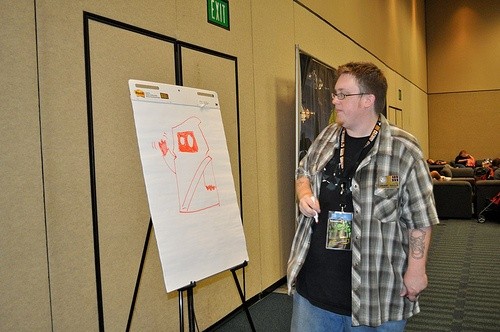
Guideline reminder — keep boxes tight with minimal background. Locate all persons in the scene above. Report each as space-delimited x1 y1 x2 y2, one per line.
426 150 500 184
287 61 440 331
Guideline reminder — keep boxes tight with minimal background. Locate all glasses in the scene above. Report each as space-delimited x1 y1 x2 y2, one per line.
331 91 370 100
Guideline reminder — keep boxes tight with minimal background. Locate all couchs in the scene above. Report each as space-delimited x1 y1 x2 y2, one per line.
427 160 499 219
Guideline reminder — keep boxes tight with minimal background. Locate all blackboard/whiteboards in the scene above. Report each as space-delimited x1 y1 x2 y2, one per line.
127 77 250 296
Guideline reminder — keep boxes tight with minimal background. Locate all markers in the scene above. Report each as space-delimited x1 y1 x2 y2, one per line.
310 195 319 224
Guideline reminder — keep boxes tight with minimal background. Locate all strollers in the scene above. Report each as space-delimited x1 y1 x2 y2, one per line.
476 191 500 224
